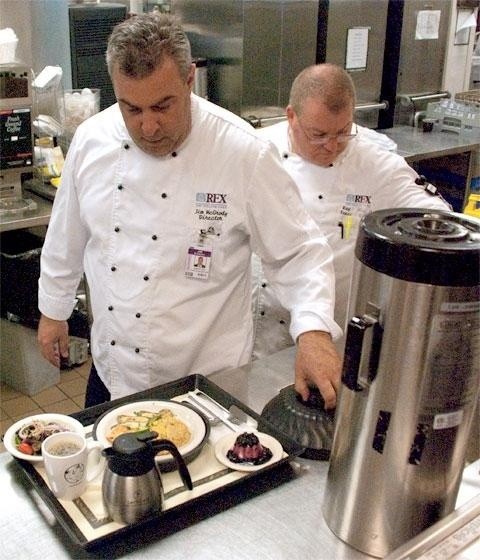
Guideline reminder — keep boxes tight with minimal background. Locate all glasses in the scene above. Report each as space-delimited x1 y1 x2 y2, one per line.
294 111 358 145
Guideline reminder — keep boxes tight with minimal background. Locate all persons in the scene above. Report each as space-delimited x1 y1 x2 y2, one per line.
37 14 343 408
249 62 457 364
194 257 205 268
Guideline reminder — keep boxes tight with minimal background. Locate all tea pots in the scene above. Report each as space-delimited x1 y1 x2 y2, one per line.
100 430 193 524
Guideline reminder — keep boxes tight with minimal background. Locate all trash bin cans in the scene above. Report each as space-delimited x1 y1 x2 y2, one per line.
0 231 62 397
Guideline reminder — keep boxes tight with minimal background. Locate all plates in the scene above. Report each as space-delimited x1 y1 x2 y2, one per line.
214 429 285 471
91 398 210 465
3 412 86 462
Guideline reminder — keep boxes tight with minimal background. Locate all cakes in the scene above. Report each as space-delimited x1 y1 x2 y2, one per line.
226 431 273 465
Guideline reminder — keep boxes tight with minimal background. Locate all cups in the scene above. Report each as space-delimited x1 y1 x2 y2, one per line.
42 431 106 500
